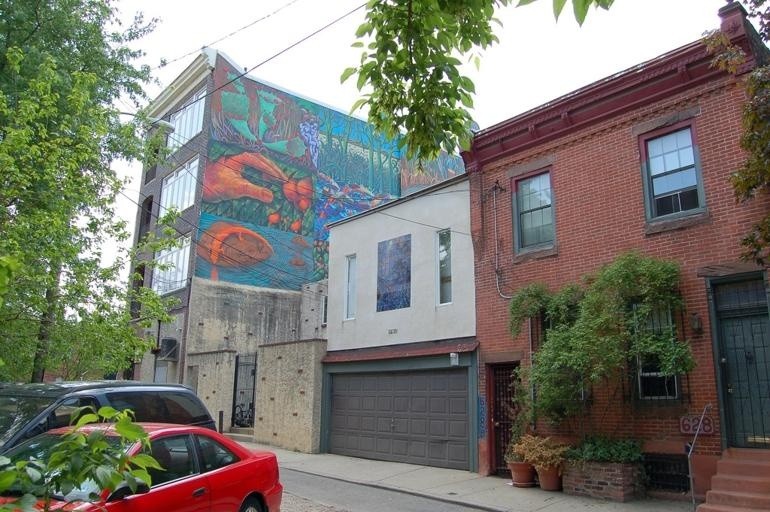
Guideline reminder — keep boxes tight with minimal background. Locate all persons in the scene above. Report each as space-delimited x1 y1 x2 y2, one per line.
202 150 289 205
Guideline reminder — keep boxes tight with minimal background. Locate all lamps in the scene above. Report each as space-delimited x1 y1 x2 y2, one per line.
688 312 704 338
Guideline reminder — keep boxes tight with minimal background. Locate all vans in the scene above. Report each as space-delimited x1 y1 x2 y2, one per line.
1 376 219 474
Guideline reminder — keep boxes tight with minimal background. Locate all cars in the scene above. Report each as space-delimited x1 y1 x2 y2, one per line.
1 421 288 511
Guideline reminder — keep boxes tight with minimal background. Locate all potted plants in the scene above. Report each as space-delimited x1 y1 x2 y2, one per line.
502 439 536 488
517 434 562 490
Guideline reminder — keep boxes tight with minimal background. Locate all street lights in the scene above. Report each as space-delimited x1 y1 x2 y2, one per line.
34 108 183 383
